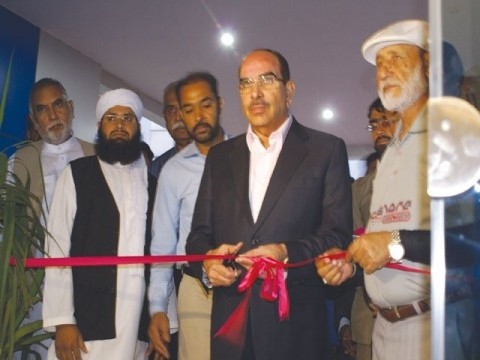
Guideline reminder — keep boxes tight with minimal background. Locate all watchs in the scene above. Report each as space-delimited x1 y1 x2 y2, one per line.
388 231 405 263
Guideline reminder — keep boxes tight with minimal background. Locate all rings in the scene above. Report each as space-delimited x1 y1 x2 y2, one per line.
322 276 327 284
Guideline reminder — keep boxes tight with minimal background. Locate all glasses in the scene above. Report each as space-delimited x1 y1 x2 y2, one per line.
104 113 136 123
238 76 285 88
369 117 399 132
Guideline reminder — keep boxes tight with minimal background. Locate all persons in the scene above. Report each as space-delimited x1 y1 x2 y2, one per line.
6 79 95 360
333 98 400 360
185 49 353 360
149 81 193 181
146 71 230 360
315 20 480 360
43 88 158 360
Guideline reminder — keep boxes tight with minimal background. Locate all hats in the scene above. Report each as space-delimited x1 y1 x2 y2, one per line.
361 18 429 66
96 88 144 123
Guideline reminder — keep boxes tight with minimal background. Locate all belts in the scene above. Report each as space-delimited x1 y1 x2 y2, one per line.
378 298 429 322
182 266 191 274
